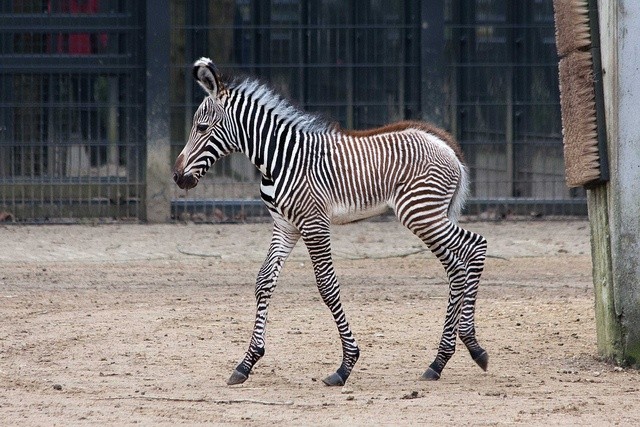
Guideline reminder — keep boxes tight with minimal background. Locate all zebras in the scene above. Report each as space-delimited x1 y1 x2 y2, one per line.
173 56 490 388
